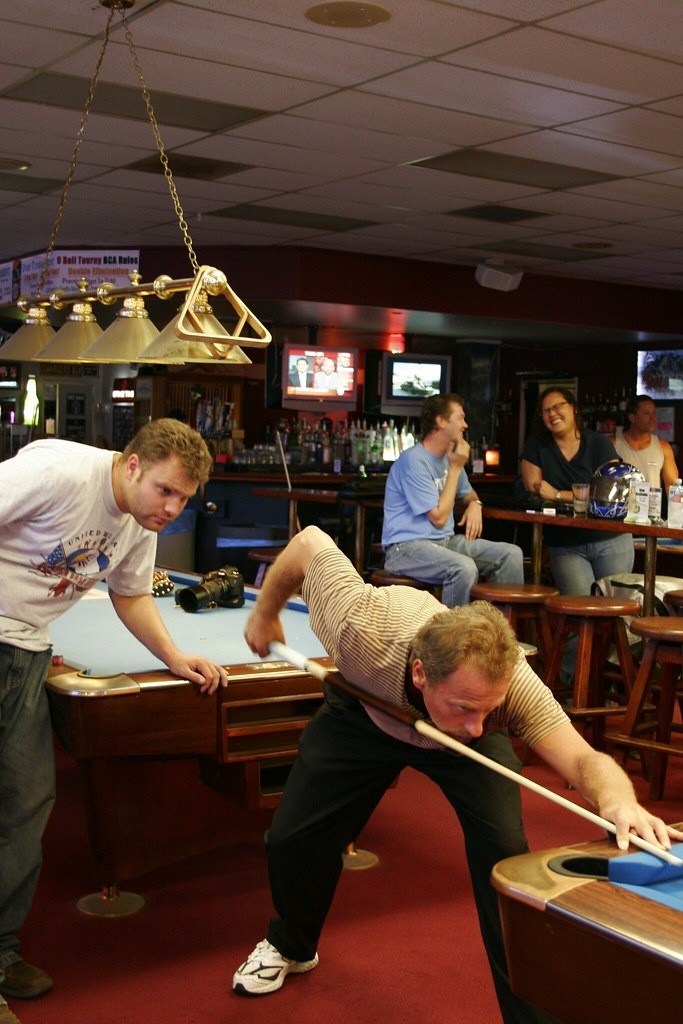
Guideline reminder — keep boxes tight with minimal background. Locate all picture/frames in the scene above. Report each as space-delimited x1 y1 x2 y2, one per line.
0 361 22 391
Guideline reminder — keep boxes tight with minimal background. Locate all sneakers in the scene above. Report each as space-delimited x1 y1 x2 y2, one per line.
232 936 319 997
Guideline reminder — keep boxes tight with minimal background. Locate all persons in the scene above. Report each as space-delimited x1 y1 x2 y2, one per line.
231 526 683 1024
413 373 425 390
519 386 635 708
381 393 537 656
288 358 314 388
314 359 338 388
0 418 229 999
608 395 678 496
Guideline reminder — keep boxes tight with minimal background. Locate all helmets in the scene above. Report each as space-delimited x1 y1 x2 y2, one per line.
589 461 645 519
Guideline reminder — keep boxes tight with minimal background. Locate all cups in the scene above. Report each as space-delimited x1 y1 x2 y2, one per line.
571 483 589 519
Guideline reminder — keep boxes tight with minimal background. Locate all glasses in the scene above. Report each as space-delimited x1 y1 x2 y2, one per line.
539 401 568 417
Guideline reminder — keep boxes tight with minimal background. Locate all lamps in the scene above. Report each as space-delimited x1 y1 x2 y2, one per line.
77 297 186 365
0 307 57 362
472 264 525 291
27 303 105 364
130 290 253 364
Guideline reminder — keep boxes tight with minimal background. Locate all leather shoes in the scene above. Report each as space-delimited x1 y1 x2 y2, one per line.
0 949 53 1024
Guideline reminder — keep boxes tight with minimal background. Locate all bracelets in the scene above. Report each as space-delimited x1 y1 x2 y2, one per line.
468 499 483 505
555 489 561 501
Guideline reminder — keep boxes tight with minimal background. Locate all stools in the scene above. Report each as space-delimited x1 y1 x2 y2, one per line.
369 568 433 592
542 594 656 792
247 546 287 587
605 614 683 803
663 588 683 735
471 581 567 707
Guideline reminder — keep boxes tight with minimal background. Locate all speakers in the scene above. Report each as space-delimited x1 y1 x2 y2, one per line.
475 264 522 291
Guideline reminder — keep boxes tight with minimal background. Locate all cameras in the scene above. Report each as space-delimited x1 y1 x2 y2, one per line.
174 567 245 614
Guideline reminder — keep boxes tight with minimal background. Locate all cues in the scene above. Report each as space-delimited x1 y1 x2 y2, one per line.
277 430 302 532
267 640 683 868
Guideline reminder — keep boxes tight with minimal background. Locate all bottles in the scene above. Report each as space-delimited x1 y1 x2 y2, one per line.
275 417 420 466
667 479 683 530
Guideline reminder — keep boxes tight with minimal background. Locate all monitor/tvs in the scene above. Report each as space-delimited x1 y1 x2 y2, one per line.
631 345 683 409
379 351 452 417
281 343 357 413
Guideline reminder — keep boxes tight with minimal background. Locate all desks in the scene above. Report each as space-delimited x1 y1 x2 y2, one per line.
349 498 683 652
39 566 380 920
253 484 355 544
492 822 683 1024
